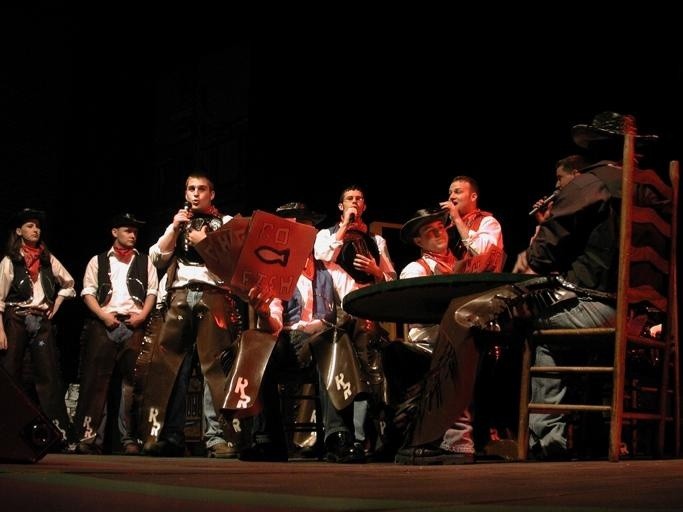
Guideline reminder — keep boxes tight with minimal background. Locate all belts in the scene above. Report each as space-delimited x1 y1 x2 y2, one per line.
168 282 228 295
115 314 130 321
13 307 46 316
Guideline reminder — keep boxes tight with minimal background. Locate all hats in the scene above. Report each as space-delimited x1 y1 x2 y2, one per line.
570 111 661 152
8 208 44 226
400 208 450 237
97 210 147 238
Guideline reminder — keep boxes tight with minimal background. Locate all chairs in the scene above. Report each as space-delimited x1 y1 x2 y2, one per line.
270 369 325 462
517 128 683 462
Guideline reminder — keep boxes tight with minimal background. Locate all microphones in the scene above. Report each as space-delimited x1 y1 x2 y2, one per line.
528 190 559 216
178 201 191 234
348 213 355 224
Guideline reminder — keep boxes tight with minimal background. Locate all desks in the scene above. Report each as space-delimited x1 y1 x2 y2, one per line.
342 271 544 462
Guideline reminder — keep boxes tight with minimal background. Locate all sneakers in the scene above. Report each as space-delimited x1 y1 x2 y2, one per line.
206 440 238 458
124 441 141 457
141 438 182 457
396 443 474 467
526 441 569 463
318 437 378 463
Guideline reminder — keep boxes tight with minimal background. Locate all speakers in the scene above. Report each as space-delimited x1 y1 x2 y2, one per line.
0 363 64 464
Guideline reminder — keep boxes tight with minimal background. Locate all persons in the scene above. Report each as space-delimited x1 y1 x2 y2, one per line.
397 203 512 463
387 106 676 464
246 201 366 460
311 183 397 468
130 169 244 460
437 176 501 276
0 207 77 452
78 211 160 457
524 154 587 242
